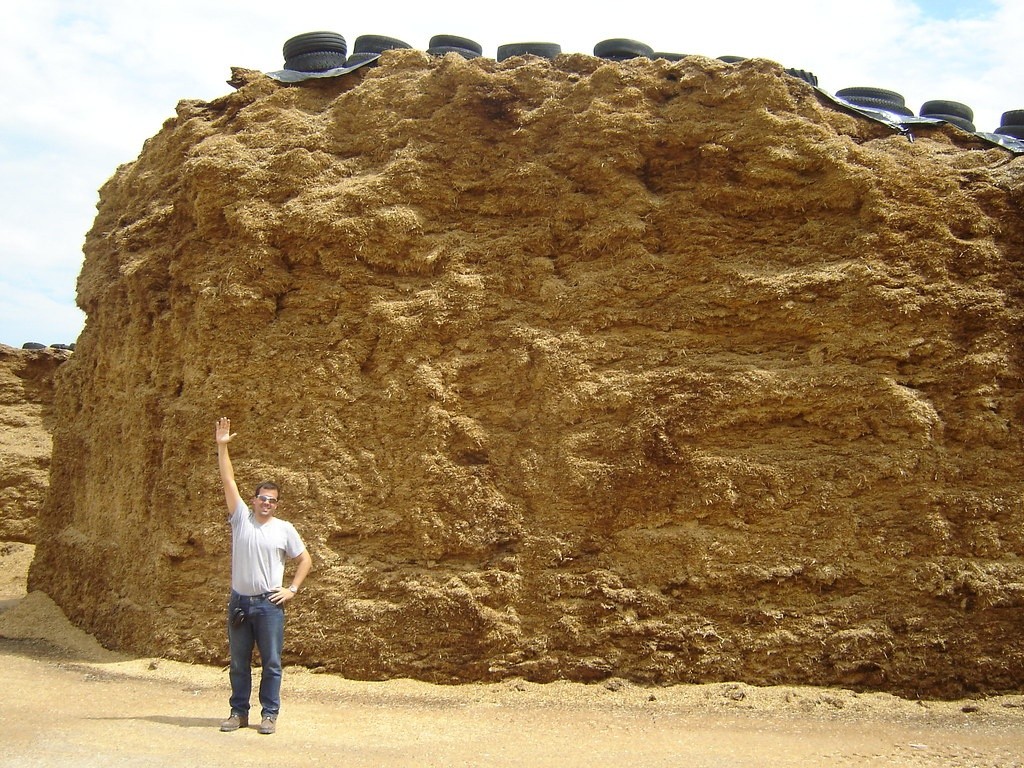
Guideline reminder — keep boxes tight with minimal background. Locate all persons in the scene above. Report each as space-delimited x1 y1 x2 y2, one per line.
216 416 312 734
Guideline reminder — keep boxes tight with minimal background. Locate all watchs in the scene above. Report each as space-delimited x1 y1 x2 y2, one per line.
289 586 298 593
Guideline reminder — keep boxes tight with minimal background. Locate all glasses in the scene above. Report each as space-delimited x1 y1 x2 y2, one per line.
257 495 278 504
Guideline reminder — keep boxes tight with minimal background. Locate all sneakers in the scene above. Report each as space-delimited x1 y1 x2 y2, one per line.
258 713 279 733
220 708 249 731
